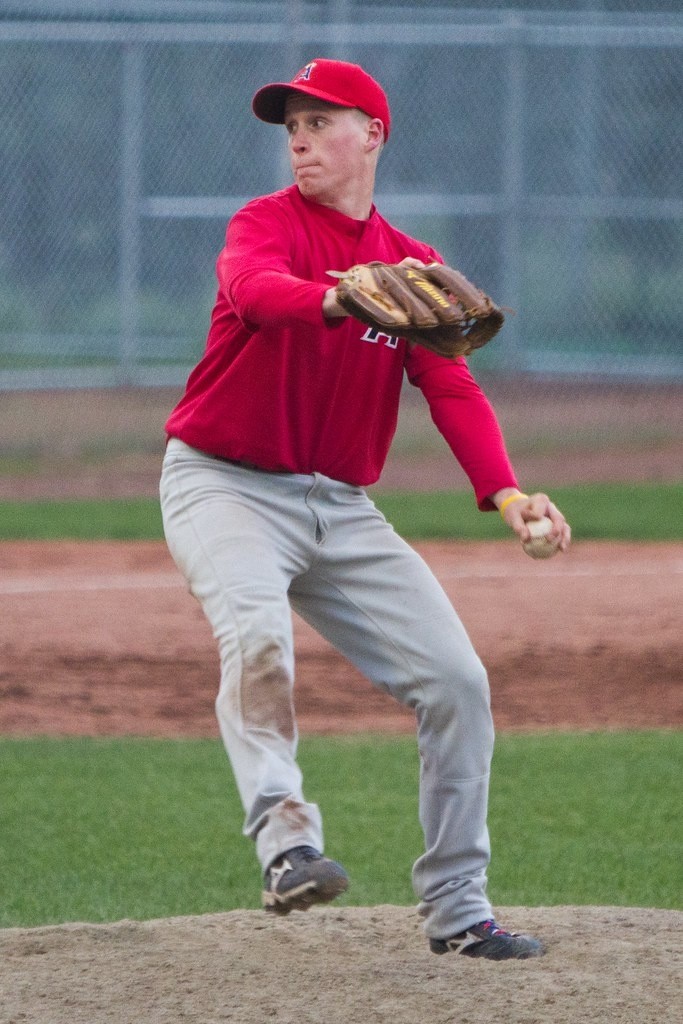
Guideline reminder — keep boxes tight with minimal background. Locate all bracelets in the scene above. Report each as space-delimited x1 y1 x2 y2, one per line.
499 492 528 520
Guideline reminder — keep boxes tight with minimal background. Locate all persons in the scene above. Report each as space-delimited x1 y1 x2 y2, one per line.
159 59 572 961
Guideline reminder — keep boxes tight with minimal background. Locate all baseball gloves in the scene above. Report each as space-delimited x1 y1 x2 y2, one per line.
333 259 506 360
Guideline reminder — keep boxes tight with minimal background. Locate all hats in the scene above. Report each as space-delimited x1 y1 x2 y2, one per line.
250 58 392 143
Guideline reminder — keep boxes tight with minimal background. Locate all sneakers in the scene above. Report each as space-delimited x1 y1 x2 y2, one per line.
259 845 350 917
428 919 545 961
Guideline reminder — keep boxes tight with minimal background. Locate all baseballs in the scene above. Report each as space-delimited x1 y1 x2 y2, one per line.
520 516 562 560
